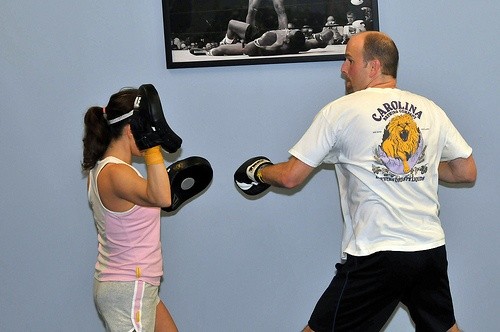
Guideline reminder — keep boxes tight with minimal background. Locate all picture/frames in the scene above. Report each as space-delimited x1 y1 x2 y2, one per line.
161 0 380 70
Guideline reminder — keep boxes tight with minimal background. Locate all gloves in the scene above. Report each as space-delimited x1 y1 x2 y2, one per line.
233 155 273 198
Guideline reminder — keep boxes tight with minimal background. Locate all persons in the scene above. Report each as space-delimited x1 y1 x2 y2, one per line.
247 1 290 32
190 20 335 57
82 84 214 332
234 32 477 332
167 6 378 50
